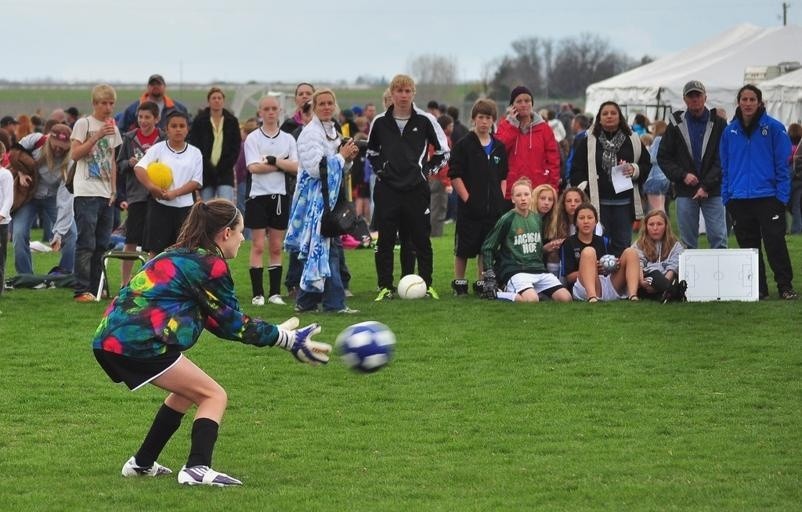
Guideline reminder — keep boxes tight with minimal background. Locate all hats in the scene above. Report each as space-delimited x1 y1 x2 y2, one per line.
509 86 533 106
683 81 706 96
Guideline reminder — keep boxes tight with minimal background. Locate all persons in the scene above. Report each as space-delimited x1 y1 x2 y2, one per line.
93 198 334 486
1 74 802 312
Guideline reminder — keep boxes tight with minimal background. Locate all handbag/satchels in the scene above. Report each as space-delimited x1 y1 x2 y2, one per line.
320 200 358 238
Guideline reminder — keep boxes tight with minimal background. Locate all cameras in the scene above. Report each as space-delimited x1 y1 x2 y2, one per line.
303 99 311 112
341 137 368 153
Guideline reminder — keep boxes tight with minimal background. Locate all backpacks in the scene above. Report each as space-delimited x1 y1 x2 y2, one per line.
65 118 90 194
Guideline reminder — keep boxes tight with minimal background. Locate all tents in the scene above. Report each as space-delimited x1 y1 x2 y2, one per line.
750 70 802 128
584 25 801 122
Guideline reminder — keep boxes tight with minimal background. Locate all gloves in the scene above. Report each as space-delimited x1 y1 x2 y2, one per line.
483 277 498 300
274 318 331 367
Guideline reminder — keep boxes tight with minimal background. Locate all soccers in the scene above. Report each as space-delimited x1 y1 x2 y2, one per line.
398 274 427 300
600 254 618 275
146 162 172 189
335 321 397 372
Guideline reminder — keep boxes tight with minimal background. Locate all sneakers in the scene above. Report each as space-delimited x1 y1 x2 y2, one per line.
451 279 468 296
269 294 288 305
177 464 243 486
122 457 172 477
472 280 485 297
660 279 688 305
780 289 797 300
426 287 441 300
74 292 96 302
326 307 360 315
373 288 394 302
294 304 323 317
252 296 265 305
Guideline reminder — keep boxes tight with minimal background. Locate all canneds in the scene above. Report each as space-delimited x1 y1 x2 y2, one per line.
106 117 115 127
